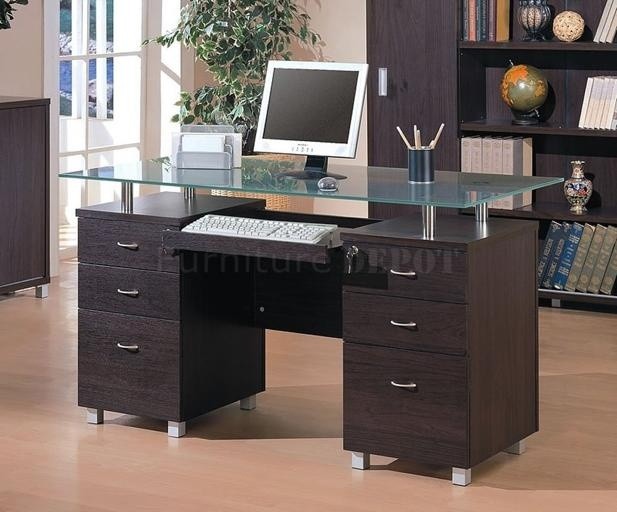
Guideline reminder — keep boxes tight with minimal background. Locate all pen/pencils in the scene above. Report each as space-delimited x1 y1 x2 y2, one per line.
396 123 446 149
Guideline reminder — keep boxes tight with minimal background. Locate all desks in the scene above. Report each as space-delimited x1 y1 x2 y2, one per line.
59 155 564 489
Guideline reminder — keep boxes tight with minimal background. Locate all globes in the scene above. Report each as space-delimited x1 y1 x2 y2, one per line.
500 64 549 126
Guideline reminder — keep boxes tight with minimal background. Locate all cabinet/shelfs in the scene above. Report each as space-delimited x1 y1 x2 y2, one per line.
363 1 617 311
0 94 52 299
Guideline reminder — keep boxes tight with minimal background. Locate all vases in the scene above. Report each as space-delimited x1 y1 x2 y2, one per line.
561 160 594 218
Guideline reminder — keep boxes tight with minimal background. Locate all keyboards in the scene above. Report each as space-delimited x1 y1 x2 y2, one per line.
181 214 344 248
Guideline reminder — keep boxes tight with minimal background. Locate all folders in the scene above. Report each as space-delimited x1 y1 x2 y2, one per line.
461 135 533 211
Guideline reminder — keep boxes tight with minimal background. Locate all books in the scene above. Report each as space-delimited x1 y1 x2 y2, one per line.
457 135 535 210
591 0 616 44
575 76 616 130
461 1 511 42
537 217 616 294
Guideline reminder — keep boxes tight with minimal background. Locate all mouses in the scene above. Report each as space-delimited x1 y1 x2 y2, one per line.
318 177 338 192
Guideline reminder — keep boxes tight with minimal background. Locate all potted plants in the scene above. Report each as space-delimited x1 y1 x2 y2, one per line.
140 1 331 213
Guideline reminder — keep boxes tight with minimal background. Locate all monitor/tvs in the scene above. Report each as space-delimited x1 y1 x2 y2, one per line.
253 60 369 181
